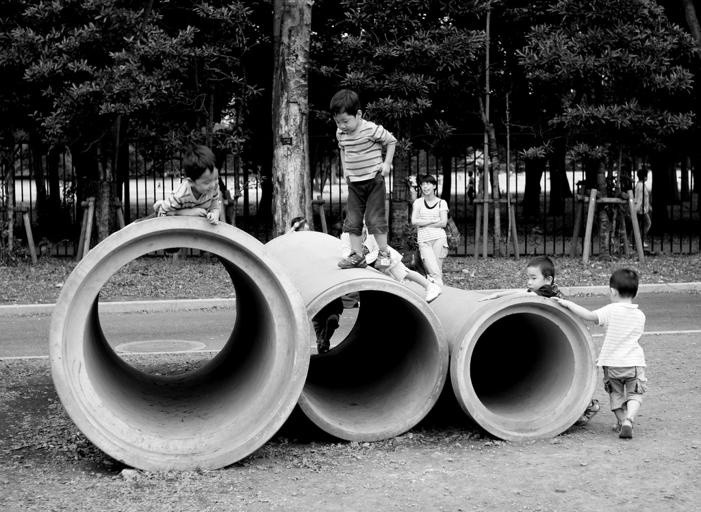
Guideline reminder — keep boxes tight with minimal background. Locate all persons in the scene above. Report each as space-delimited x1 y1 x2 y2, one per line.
313 297 345 356
465 171 477 205
634 166 653 248
548 269 648 440
290 217 309 233
477 256 601 428
328 89 398 271
411 173 451 281
342 206 443 303
153 145 223 224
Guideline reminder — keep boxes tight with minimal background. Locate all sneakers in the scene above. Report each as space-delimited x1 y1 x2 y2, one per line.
338 250 368 268
375 250 392 269
576 400 599 425
612 418 633 438
425 282 441 303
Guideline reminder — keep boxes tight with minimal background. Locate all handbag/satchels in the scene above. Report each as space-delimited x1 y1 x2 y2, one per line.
445 216 462 249
